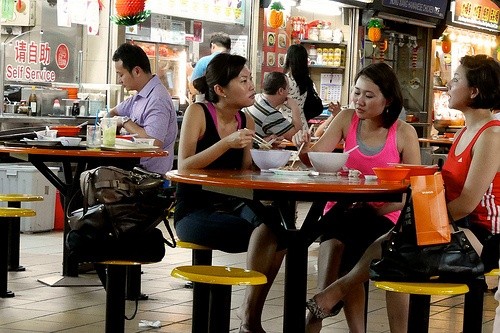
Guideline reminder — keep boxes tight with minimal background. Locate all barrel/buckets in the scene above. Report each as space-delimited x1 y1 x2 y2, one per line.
3 104 19 113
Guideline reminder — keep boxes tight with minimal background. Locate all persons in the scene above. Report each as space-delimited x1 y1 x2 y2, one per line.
189 32 231 95
314 99 405 137
241 71 303 229
283 44 318 220
174 53 287 333
306 54 500 333
291 62 421 333
78 42 179 274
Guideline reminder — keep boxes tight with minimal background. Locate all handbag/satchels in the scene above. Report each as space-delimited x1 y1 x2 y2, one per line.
368 197 484 284
304 87 324 121
410 173 451 246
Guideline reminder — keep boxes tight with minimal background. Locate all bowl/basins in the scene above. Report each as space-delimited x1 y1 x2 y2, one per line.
307 151 350 175
249 149 291 173
397 166 440 180
34 125 83 146
371 168 411 185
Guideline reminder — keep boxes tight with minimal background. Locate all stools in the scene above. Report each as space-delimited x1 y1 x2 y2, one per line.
171 266 268 333
101 261 140 333
463 269 500 333
374 281 469 333
175 241 214 333
0 193 44 272
0 207 36 298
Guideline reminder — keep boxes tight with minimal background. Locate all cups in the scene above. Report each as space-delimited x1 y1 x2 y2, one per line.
87 125 101 152
64 106 72 116
101 117 118 147
90 100 105 116
78 100 90 117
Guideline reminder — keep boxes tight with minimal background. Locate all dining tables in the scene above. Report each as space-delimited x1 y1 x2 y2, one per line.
418 136 453 168
0 145 169 287
163 168 410 333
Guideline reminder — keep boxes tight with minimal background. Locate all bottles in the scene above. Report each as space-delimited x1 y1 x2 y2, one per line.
72 102 80 117
29 86 37 116
308 25 343 58
52 99 61 116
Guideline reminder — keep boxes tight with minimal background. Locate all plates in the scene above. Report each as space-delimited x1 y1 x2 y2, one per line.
364 175 377 180
20 139 60 146
81 141 160 152
269 169 311 176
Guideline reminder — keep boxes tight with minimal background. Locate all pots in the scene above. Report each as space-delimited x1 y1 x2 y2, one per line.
431 117 465 135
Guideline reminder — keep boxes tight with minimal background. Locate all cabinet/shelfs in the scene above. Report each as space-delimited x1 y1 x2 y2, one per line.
298 41 346 122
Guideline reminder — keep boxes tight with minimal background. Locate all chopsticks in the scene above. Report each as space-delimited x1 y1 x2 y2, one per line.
291 125 314 167
243 128 273 149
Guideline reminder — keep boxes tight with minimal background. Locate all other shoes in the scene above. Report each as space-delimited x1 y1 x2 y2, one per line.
239 322 265 333
306 293 343 318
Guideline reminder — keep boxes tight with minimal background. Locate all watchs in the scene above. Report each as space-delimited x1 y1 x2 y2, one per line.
122 116 131 127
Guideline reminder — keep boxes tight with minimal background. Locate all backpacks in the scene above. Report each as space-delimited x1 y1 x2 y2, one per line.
66 166 176 262
